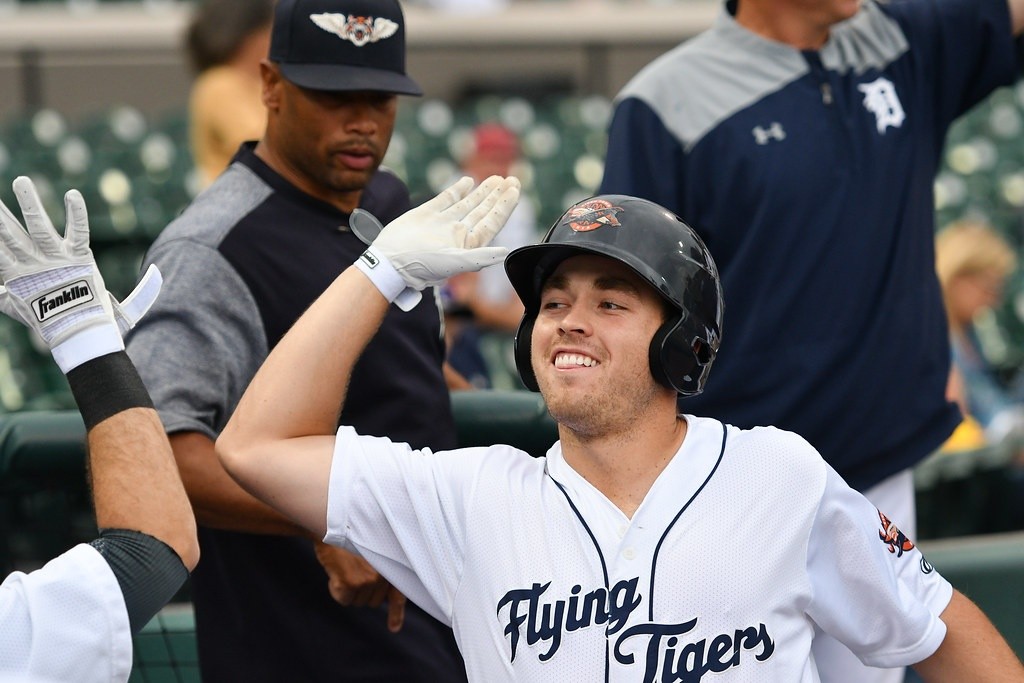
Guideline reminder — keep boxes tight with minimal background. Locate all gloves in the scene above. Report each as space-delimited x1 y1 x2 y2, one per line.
0 176 164 375
356 173 520 310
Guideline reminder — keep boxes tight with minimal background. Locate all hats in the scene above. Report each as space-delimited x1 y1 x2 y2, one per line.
268 0 424 97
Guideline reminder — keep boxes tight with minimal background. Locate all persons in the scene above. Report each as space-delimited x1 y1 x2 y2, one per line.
601 0 1024 683
216 174 1024 683
0 175 201 683
127 0 532 683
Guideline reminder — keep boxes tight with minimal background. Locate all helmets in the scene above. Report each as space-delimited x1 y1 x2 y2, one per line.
505 195 721 398
